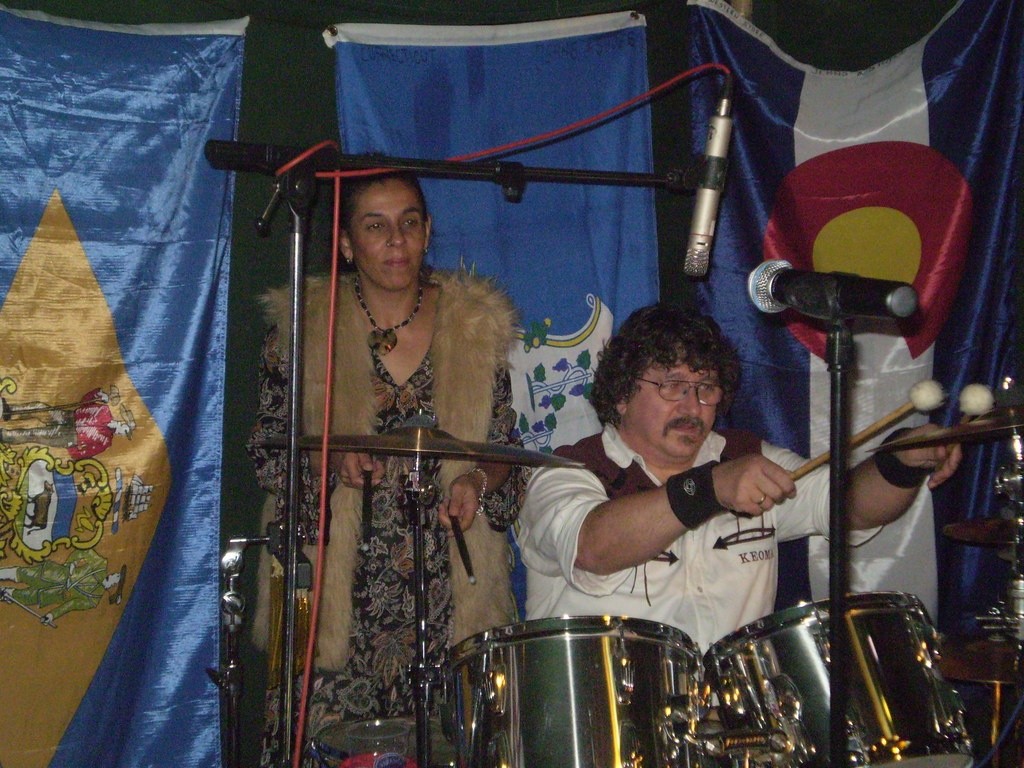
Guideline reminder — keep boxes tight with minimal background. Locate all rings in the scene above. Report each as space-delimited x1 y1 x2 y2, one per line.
758 494 766 504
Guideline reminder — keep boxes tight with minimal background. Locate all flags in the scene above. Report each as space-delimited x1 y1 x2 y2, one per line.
2 1 1020 768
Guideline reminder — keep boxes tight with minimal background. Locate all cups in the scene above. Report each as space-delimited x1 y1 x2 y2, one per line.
347 719 411 768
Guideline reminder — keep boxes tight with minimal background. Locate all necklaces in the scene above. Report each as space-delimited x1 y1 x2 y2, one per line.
356 273 423 356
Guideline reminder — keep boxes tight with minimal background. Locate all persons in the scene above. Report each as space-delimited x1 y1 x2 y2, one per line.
248 170 520 768
512 300 961 768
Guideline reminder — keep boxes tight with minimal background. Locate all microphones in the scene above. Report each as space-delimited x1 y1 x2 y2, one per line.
745 259 921 320
685 74 735 275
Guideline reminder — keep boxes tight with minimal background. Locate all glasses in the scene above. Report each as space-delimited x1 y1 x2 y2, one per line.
637 378 725 406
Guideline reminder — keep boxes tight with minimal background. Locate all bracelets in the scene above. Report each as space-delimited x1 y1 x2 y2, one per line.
875 427 923 488
666 461 727 530
469 466 487 516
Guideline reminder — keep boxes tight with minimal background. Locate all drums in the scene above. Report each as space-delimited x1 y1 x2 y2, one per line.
444 616 709 768
703 589 973 768
311 717 458 768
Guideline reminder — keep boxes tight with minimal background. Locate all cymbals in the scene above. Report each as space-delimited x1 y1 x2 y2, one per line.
939 637 1024 685
940 516 1023 545
867 403 1024 456
263 427 593 471
